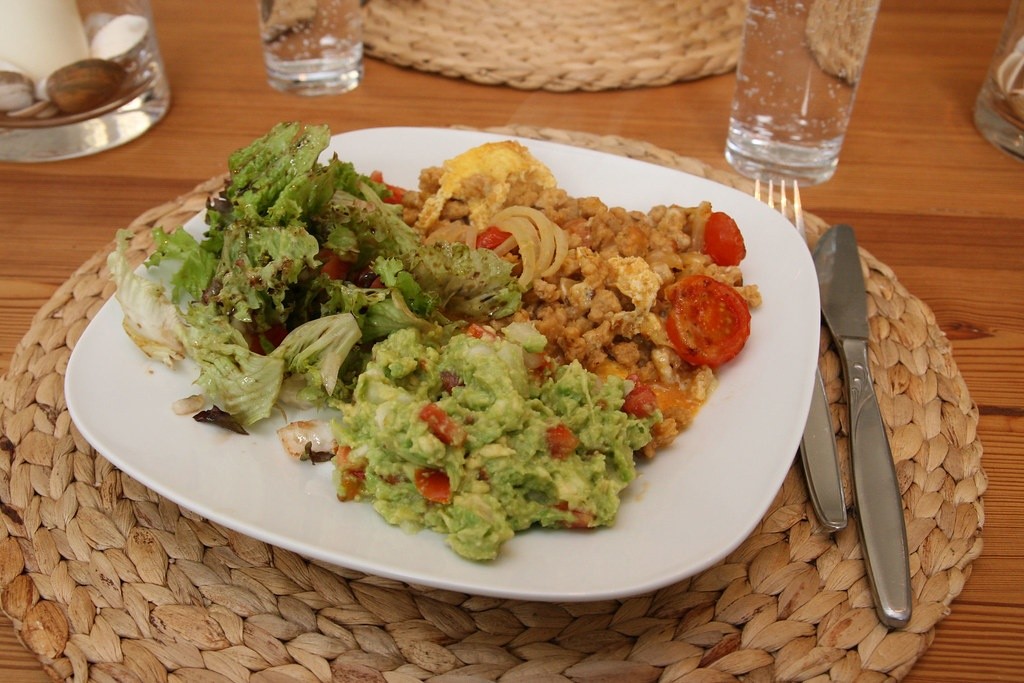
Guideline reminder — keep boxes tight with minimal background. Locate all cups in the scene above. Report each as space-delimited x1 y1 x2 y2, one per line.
0 1 171 162
724 0 879 186
256 0 364 96
972 0 1024 162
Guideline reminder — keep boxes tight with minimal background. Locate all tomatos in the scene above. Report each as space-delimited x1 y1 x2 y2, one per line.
704 210 747 269
668 276 751 368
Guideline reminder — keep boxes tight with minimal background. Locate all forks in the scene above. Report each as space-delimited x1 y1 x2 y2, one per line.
750 178 849 528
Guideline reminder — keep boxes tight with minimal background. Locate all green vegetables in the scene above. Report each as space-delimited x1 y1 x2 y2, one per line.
108 120 525 434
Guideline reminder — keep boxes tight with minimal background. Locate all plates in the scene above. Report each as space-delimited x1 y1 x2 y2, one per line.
62 125 822 598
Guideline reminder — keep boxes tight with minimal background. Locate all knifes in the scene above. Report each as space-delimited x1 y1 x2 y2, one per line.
812 225 912 626
